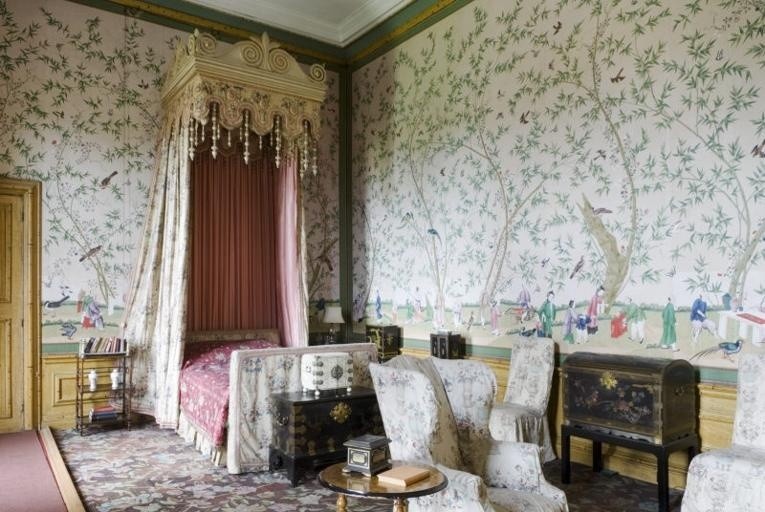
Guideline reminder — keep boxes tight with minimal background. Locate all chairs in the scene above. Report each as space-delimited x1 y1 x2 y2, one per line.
369 355 569 512
680 341 765 512
489 336 556 462
431 356 497 441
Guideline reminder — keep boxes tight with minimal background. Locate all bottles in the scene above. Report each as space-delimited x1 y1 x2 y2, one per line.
88 369 98 391
111 368 119 390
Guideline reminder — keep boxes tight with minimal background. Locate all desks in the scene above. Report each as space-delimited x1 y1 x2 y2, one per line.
560 424 700 511
319 459 448 512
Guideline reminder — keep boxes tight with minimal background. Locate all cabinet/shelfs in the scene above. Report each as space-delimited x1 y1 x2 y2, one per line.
75 354 132 436
269 387 377 488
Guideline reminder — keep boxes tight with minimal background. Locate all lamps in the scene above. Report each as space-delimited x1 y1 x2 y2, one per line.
323 306 345 344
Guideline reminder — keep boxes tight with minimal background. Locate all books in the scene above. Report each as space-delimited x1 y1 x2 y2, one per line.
378 465 432 487
85 337 128 353
88 406 119 421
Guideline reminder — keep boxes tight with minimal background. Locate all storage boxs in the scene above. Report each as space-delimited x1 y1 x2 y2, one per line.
562 352 698 444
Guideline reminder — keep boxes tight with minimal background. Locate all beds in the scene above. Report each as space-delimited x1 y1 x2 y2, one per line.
122 327 379 475
